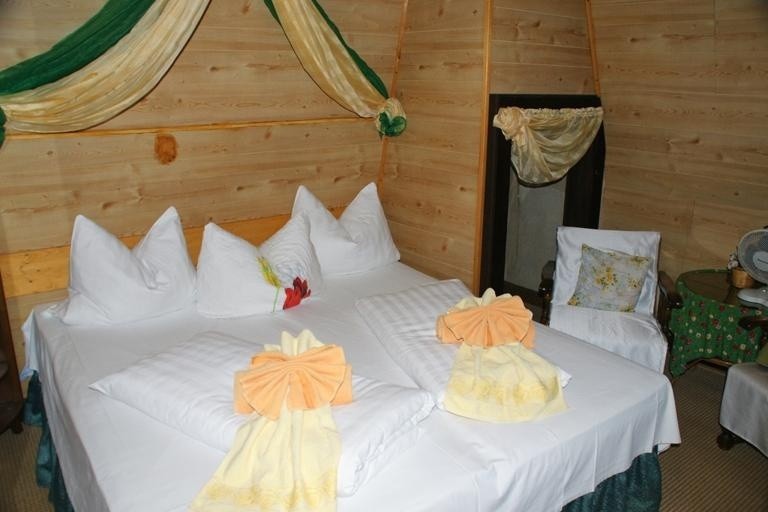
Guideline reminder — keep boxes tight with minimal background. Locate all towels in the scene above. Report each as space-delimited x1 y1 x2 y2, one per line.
434 286 571 426
185 323 356 512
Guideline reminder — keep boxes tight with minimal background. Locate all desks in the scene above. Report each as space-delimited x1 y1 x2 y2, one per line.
678 268 768 360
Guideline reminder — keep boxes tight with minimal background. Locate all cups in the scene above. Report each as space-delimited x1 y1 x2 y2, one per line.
733 267 753 289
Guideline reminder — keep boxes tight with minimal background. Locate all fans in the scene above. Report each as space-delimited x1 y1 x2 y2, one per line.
733 227 768 309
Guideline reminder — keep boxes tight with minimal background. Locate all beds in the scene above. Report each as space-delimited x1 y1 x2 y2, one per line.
25 263 672 512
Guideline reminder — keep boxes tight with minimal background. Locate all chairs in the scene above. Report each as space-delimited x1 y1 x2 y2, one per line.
537 226 683 372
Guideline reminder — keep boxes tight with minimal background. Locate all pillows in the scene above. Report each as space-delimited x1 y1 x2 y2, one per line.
197 212 325 321
289 184 403 283
566 241 653 312
61 205 198 325
